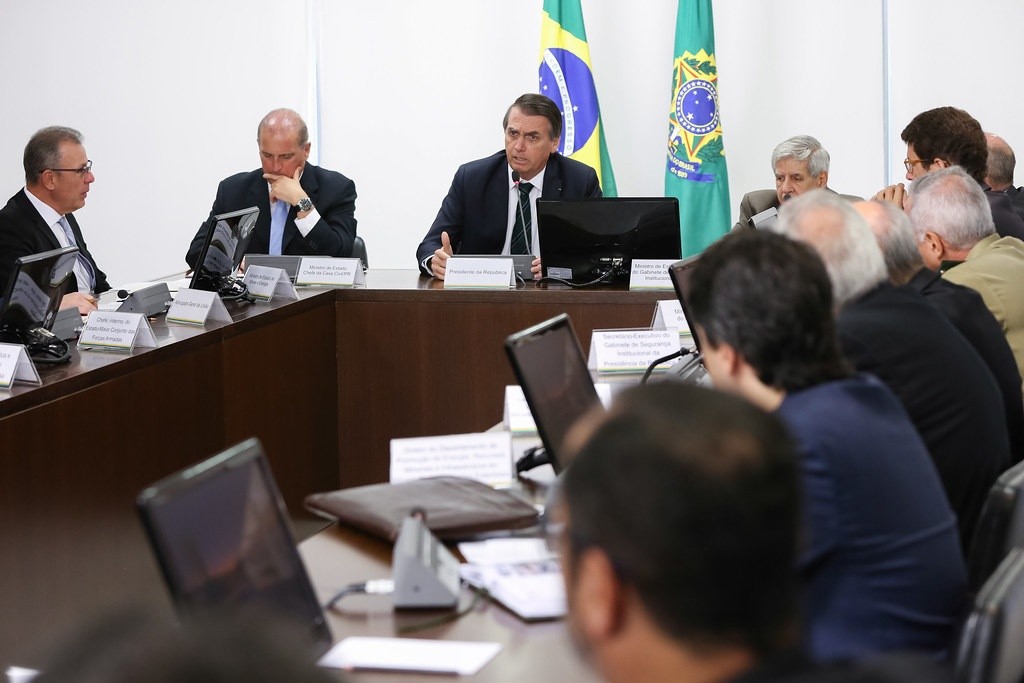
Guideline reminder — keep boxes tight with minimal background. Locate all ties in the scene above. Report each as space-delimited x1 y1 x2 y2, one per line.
510 182 534 255
269 200 288 257
58 221 95 292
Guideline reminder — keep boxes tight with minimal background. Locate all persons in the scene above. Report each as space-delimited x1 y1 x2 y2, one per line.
549 379 835 683
731 135 863 230
688 226 971 683
778 189 1009 534
986 133 1024 220
855 199 1024 459
186 109 357 272
416 93 604 282
873 106 1024 241
1 126 111 315
29 603 339 683
906 166 1024 396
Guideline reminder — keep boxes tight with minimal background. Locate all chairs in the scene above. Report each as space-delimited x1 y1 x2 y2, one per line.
959 460 1024 591
951 549 1024 683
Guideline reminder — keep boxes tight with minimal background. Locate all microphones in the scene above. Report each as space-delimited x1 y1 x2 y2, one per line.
513 170 533 256
784 194 791 201
117 290 131 299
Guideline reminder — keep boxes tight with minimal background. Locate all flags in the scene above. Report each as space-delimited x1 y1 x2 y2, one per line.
666 0 731 257
538 0 617 196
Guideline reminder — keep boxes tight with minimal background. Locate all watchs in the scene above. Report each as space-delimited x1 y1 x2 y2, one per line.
293 198 312 212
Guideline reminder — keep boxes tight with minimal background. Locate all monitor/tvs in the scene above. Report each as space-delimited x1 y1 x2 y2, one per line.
0 245 80 354
535 197 683 288
747 207 781 235
135 436 334 665
668 250 708 372
503 313 607 479
189 206 260 297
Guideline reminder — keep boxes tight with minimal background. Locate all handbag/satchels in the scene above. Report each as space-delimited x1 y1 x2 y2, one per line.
306 475 539 546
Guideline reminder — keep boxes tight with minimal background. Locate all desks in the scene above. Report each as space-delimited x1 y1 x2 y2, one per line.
0 249 710 683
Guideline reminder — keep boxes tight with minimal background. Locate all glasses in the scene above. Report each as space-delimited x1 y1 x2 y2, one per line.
38 160 93 179
904 157 949 174
